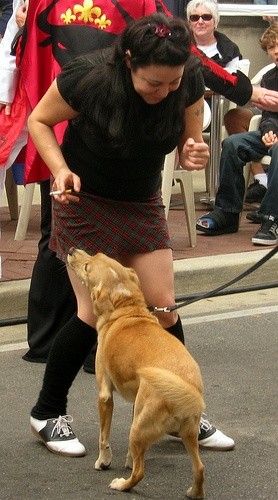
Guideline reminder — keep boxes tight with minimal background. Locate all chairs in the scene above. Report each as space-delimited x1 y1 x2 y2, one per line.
244 62 278 206
162 145 197 249
171 59 250 195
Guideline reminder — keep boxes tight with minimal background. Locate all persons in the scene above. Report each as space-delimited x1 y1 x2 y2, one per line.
0 0 278 458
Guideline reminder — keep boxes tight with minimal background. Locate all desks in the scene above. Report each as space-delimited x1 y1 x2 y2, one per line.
169 85 224 207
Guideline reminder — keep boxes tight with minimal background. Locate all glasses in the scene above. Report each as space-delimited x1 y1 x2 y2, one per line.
190 14 212 21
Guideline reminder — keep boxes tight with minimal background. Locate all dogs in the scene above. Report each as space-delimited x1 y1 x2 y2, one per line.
67 247 207 500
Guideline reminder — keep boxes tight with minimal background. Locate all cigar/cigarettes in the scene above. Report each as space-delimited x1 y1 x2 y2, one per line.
49 189 72 196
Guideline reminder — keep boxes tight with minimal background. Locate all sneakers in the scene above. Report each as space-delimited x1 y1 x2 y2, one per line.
167 412 235 451
196 210 239 235
246 208 261 224
29 415 86 457
252 215 278 245
245 179 267 203
